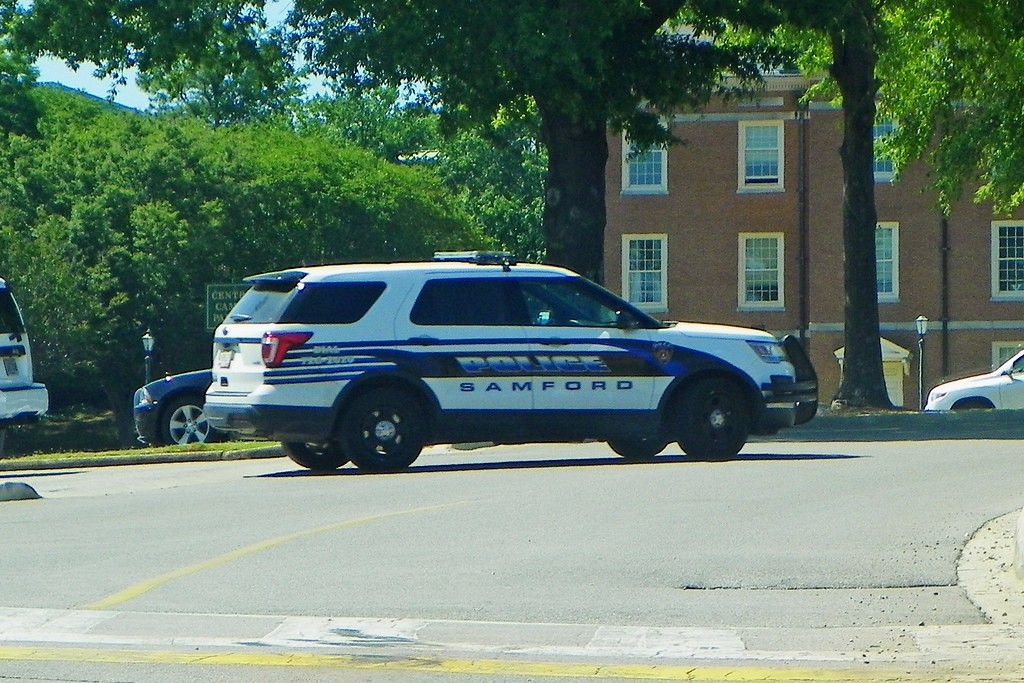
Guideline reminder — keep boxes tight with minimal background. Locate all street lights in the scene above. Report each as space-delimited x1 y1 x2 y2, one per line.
915 314 928 411
142 326 157 387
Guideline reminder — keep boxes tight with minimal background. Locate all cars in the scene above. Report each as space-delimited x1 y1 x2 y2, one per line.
925 349 1024 409
134 367 235 446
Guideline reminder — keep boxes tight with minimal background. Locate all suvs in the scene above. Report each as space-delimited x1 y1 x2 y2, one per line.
204 251 820 474
0 275 50 427
